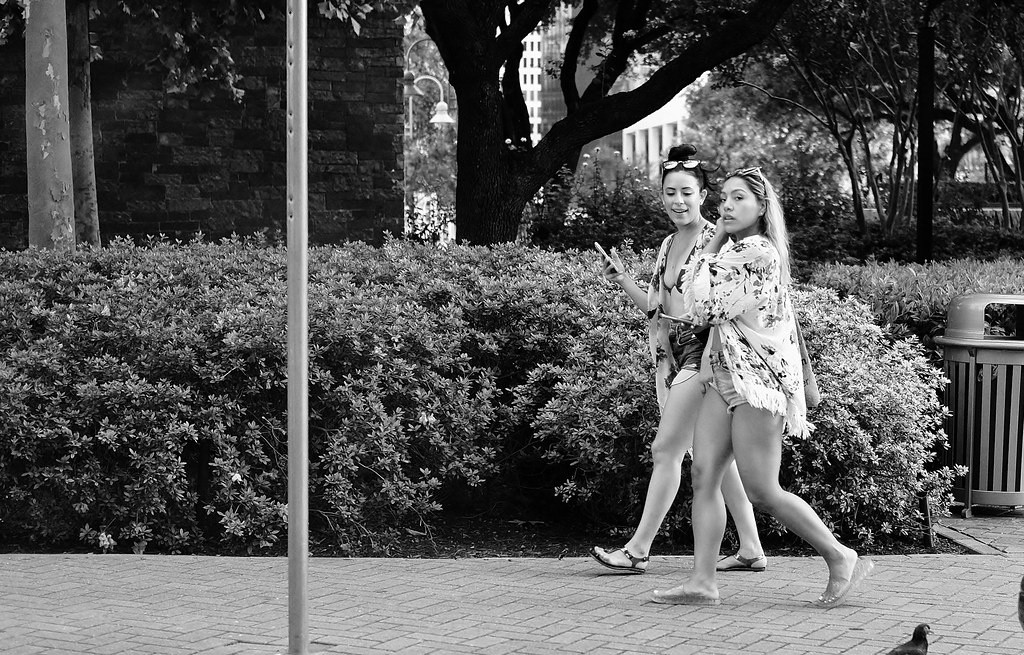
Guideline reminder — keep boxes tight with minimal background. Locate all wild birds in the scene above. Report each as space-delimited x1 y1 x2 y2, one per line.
885 623 934 655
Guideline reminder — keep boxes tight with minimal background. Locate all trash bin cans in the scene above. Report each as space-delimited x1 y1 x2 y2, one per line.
930 292 1024 508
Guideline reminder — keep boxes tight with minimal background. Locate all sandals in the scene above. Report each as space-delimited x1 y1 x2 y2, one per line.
588 546 650 573
715 552 765 572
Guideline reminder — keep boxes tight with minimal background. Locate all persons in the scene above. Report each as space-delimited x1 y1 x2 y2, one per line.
589 143 767 573
650 166 876 609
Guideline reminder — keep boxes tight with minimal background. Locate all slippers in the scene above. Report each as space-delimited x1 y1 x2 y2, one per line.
818 558 875 608
651 585 720 605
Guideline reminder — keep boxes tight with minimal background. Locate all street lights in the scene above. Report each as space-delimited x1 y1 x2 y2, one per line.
407 75 456 237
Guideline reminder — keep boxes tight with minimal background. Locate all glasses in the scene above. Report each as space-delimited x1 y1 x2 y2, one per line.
662 159 702 171
726 166 766 196
